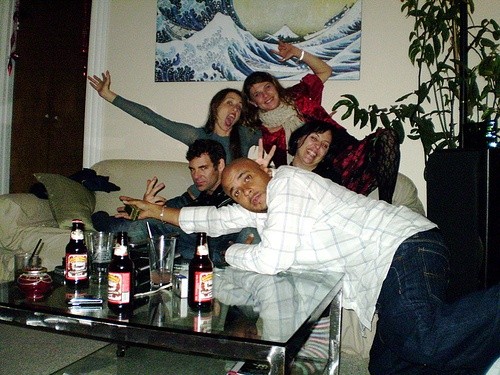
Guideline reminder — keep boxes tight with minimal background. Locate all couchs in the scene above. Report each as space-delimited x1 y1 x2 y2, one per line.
0 159 424 358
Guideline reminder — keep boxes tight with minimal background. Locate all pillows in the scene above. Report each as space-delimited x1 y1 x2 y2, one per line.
33 172 95 230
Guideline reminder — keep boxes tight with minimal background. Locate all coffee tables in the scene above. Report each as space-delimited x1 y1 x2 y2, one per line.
0 257 344 375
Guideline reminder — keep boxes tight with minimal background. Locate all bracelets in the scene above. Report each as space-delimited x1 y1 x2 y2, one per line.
298 50 304 60
160 208 165 222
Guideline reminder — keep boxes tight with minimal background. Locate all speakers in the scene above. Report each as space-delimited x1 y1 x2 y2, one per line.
426 147 500 291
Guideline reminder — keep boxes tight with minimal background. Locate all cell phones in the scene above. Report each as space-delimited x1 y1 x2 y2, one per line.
125 203 140 221
68 306 102 311
68 295 103 305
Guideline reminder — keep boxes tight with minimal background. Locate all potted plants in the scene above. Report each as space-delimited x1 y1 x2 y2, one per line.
332 0 500 290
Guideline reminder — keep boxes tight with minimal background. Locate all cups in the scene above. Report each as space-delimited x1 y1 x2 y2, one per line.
147 286 173 327
14 253 39 286
148 236 176 288
86 231 113 284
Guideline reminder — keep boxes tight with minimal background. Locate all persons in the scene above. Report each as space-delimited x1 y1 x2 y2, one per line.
114 157 500 375
87 40 401 268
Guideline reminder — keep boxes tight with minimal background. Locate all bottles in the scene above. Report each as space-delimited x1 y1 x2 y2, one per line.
189 311 212 334
107 232 135 313
16 267 53 299
187 232 214 312
65 220 88 290
